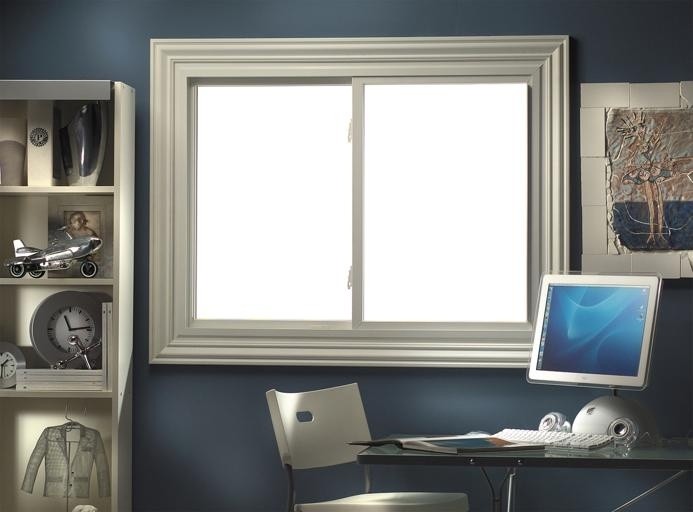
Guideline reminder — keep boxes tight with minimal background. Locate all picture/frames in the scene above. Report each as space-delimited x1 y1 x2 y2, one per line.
54 203 107 277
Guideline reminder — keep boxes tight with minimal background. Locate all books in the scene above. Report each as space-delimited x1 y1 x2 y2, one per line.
345 435 547 455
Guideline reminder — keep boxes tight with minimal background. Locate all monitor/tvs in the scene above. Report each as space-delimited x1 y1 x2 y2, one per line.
525 269 664 391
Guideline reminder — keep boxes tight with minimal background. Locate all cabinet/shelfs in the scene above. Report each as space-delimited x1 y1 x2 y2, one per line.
0 77 136 512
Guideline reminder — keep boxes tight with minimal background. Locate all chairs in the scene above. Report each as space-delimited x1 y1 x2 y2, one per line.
262 379 470 512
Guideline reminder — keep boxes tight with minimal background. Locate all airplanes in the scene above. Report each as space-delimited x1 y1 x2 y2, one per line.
5 231 104 278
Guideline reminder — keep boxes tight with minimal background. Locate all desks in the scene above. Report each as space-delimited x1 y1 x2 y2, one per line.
354 433 692 512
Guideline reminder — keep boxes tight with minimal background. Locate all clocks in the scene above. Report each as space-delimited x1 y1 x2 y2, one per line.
28 289 103 369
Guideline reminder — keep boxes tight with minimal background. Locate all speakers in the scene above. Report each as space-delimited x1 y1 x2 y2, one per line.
607 416 640 447
538 411 566 431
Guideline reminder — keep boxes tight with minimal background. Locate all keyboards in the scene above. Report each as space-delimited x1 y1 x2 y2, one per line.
490 427 614 450
